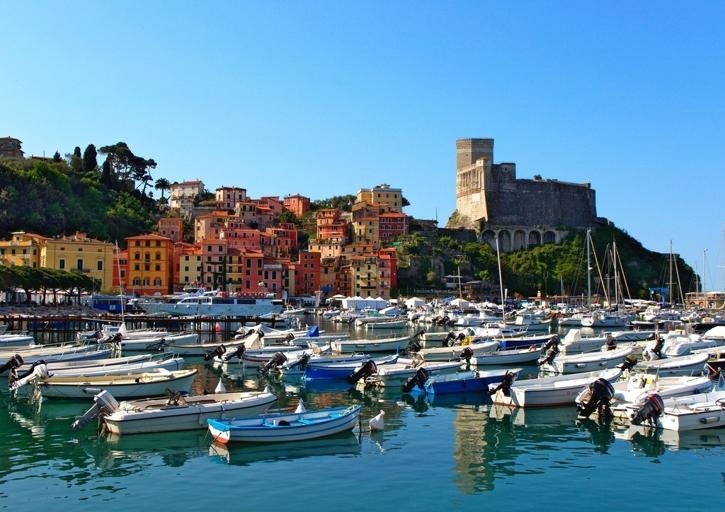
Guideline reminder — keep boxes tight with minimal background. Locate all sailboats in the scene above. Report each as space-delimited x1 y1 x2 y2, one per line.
497 227 724 324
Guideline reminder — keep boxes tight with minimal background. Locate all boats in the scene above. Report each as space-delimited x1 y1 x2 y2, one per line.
10 398 95 440
0 324 198 398
316 304 407 331
84 429 212 475
258 313 291 327
163 340 245 356
71 385 278 435
127 287 220 310
136 292 282 317
208 433 360 466
